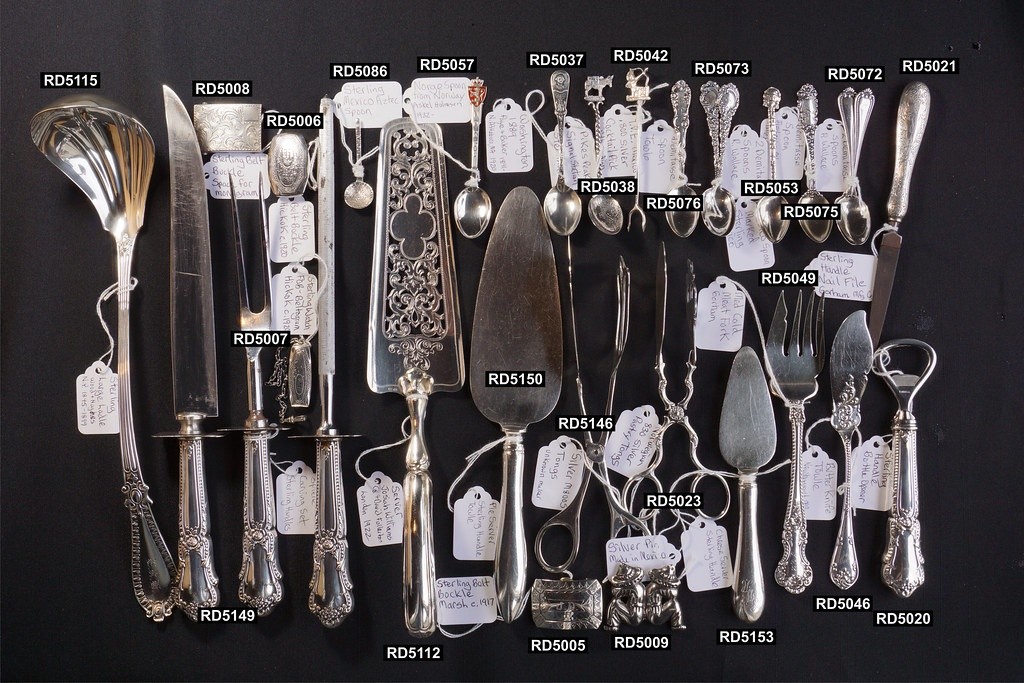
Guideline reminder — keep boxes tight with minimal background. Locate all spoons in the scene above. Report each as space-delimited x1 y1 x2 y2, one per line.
453 65 870 248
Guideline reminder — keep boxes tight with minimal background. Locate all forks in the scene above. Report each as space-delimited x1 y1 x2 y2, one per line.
763 285 828 597
227 170 285 615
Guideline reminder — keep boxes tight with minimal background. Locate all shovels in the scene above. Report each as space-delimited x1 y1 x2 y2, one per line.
364 113 468 643
717 341 780 625
468 180 566 627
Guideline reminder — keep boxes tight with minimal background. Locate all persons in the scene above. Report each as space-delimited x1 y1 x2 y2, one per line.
604 563 687 632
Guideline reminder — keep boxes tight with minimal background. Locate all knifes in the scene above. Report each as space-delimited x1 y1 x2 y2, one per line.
829 309 876 591
162 85 222 623
466 187 566 623
718 346 778 624
869 82 933 349
